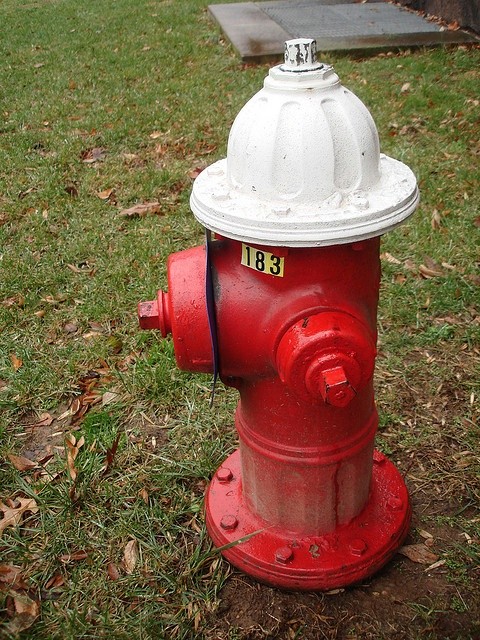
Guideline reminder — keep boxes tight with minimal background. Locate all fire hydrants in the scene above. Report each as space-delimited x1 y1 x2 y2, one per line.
136 38 420 594
